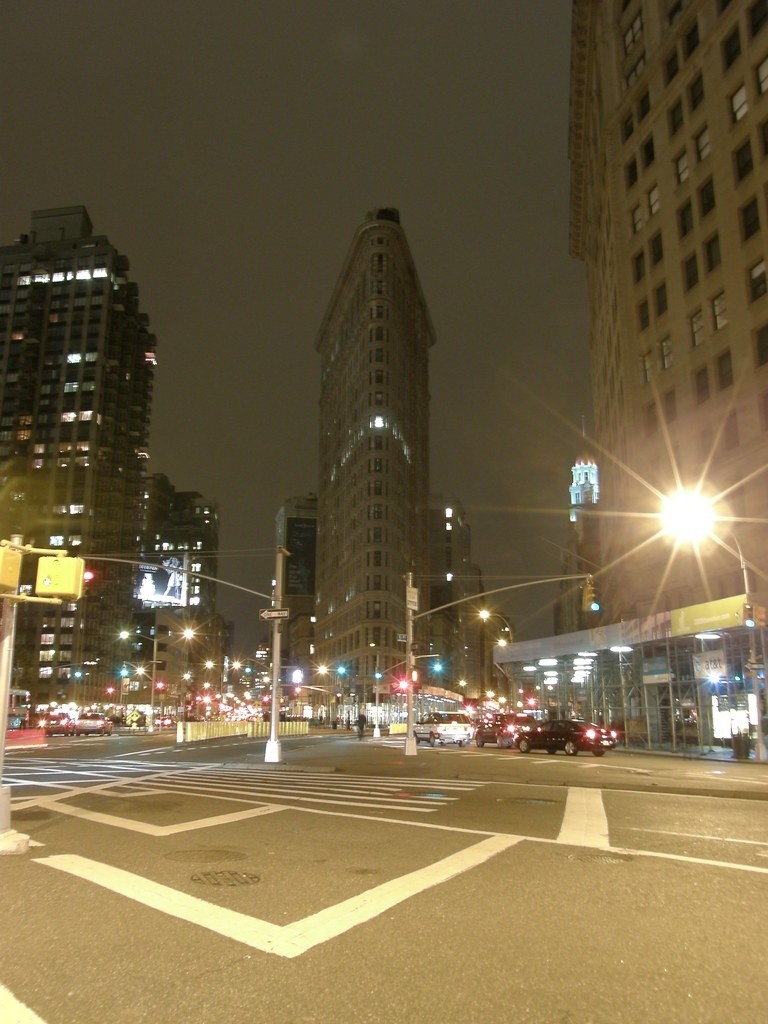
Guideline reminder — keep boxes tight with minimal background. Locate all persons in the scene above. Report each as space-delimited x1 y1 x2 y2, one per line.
346 717 351 730
162 556 183 595
357 714 366 742
22 719 26 730
320 716 323 723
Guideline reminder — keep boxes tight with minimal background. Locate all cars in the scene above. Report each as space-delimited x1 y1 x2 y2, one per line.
39 712 113 736
414 710 616 756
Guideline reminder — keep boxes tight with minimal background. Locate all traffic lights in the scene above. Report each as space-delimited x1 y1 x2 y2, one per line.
433 656 442 672
398 680 408 691
154 681 165 691
84 568 101 584
375 672 382 680
292 669 305 685
581 587 601 613
245 668 252 675
105 687 115 696
337 665 345 675
742 604 756 630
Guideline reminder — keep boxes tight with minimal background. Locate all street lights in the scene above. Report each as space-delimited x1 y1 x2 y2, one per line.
475 612 515 647
118 629 195 729
655 489 767 762
